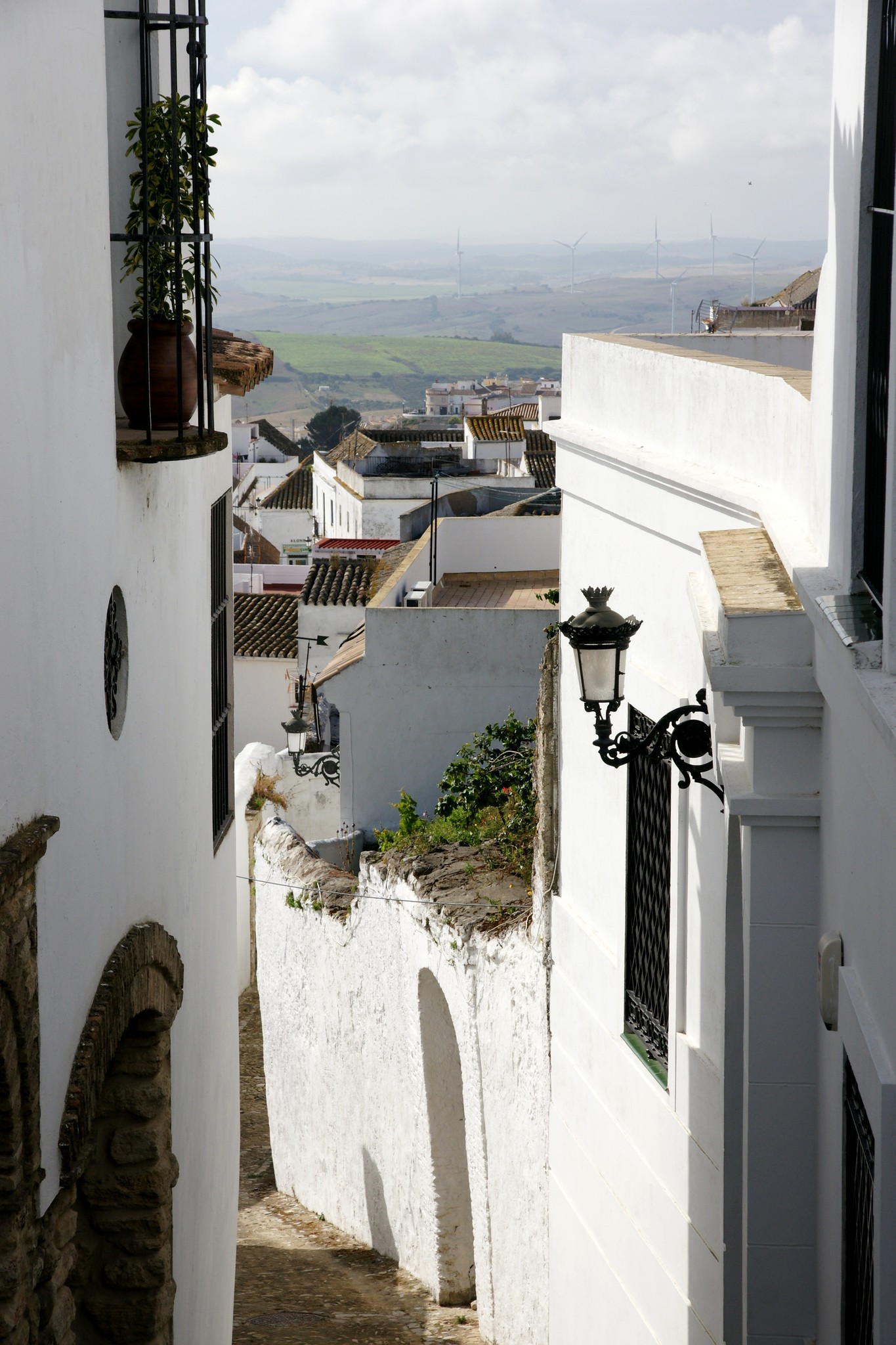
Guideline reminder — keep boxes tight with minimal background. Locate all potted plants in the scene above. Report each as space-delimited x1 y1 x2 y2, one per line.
118 92 221 430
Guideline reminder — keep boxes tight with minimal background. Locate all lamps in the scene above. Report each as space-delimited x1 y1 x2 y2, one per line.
281 710 340 789
556 586 725 814
